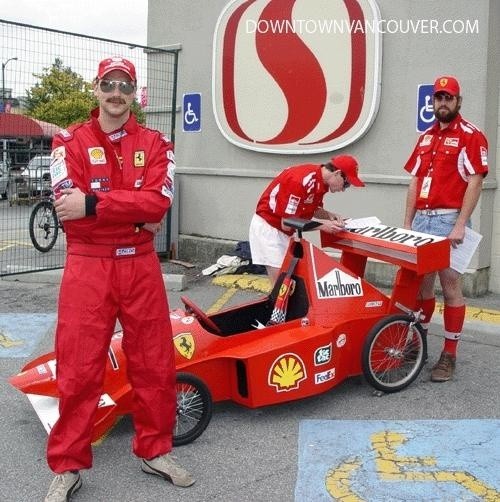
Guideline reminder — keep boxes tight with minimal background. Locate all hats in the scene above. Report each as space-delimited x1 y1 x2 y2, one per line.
434 77 460 96
98 57 136 81
333 155 366 187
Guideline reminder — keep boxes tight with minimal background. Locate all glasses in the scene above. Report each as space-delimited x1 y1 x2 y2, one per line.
343 176 350 188
100 79 135 95
435 94 454 101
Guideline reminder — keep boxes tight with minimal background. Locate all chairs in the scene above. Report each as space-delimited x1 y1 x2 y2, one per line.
179 296 224 336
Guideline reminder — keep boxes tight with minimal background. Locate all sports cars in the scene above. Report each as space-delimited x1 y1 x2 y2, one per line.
7 214 452 458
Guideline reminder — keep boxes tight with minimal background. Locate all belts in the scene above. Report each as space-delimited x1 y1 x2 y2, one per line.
418 207 461 216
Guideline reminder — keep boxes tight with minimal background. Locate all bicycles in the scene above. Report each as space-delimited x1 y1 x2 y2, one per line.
30 199 64 253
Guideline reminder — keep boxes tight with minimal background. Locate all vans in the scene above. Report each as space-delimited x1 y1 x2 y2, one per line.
20 153 51 195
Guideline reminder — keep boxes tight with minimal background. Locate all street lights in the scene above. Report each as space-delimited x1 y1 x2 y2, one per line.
1 55 18 106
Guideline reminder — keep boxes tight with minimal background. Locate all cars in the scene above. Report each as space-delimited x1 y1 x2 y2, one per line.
8 162 27 182
0 165 9 199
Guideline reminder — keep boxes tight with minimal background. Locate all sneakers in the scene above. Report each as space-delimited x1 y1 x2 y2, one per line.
431 352 456 381
405 339 428 363
44 471 82 501
141 454 195 488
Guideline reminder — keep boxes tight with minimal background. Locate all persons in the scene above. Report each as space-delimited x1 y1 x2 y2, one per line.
246 155 368 285
400 75 488 382
41 55 196 502
